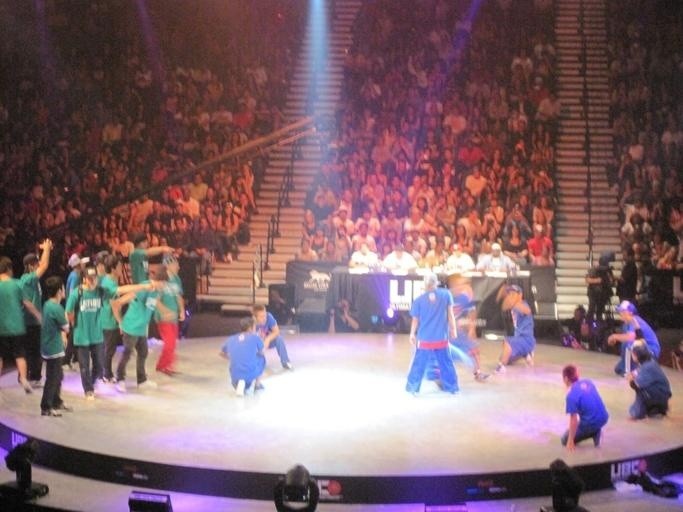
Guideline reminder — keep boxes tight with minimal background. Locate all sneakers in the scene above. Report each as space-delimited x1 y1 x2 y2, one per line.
85 390 96 402
41 408 63 417
30 380 46 389
593 427 602 448
234 379 247 397
284 360 294 371
117 380 127 394
137 380 159 391
475 371 491 383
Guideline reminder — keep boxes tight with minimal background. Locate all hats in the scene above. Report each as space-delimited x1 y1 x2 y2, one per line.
505 283 523 293
23 253 39 266
615 300 637 314
68 252 90 268
81 265 98 279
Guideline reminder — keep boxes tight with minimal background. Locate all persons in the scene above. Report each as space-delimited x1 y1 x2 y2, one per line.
300 1 560 276
614 245 637 305
607 300 659 376
624 338 672 420
446 272 493 384
606 1 683 273
268 287 296 328
325 296 361 336
0 234 191 418
561 307 602 351
2 1 311 276
217 316 266 397
493 284 536 374
413 330 489 394
403 273 460 396
585 257 615 321
248 302 292 371
558 365 609 453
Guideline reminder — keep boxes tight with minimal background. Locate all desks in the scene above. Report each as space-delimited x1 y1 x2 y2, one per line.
322 263 536 336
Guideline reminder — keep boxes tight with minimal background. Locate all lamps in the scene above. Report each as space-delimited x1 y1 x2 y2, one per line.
538 457 590 511
1 438 48 501
270 463 320 511
